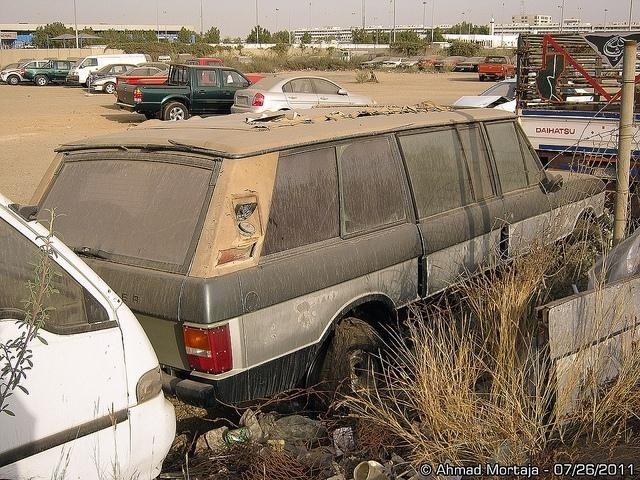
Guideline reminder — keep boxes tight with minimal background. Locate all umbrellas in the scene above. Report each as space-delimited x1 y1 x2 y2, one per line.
70 32 103 48
49 33 76 49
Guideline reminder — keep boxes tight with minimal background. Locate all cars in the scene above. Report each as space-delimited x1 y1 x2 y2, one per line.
23 102 609 406
2 48 377 125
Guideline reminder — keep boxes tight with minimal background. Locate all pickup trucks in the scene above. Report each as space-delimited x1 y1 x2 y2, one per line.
478 54 516 82
